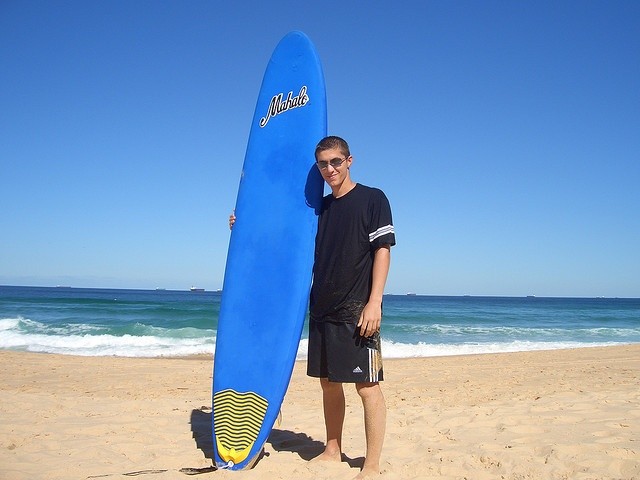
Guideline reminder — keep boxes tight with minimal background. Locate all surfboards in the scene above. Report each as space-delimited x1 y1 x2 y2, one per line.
213 29 328 470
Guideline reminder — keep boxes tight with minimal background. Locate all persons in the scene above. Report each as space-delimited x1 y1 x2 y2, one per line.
228 134 395 480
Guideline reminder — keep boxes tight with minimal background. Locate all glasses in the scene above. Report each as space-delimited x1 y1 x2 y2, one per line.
316 156 349 168
